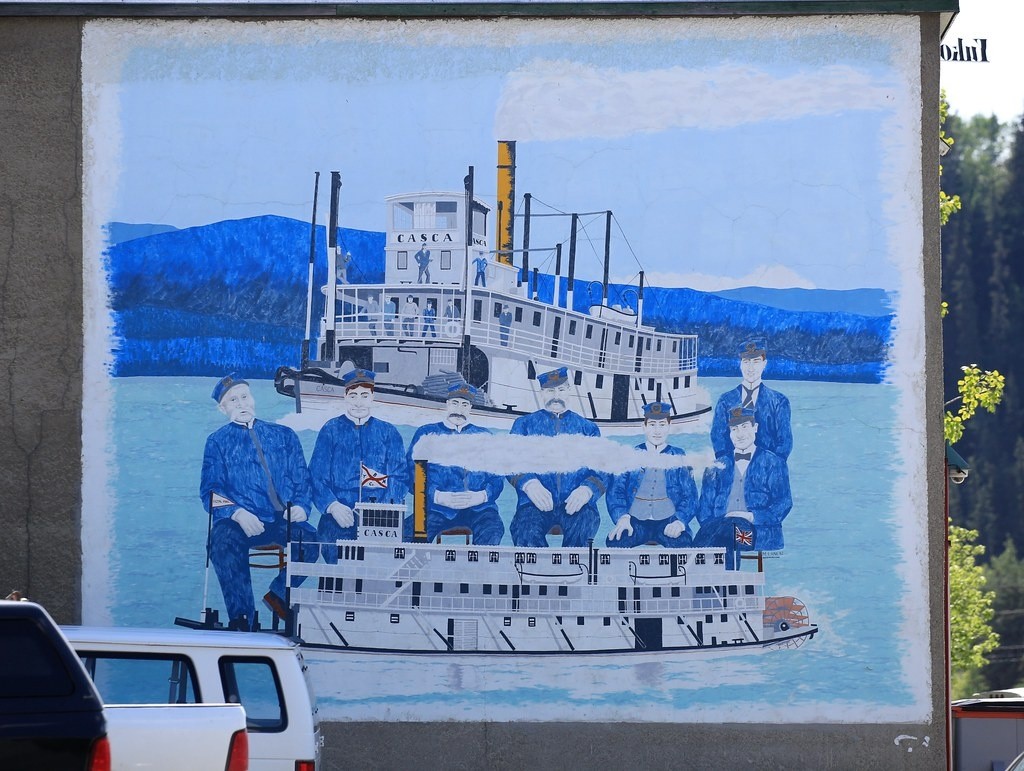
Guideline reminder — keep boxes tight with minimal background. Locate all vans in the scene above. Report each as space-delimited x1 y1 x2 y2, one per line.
60 622 322 771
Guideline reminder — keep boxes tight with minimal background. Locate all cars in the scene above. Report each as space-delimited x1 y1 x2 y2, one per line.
1 591 113 771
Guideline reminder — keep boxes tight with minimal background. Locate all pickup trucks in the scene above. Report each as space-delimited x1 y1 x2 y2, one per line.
102 701 252 771
